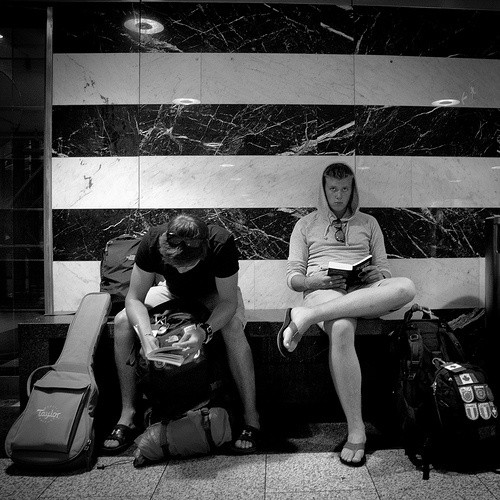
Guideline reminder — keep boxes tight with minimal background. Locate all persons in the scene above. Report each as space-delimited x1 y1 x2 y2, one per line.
99 213 266 456
276 159 416 467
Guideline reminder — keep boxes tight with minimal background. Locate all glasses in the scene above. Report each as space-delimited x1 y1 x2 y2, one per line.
165 232 203 248
332 218 345 243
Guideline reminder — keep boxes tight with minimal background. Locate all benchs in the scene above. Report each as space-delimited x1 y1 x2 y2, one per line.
19 310 441 420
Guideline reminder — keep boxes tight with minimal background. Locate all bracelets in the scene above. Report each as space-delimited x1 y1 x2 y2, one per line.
304 278 310 290
197 322 213 344
381 272 386 279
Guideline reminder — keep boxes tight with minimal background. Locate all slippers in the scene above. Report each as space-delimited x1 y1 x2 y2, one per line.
101 424 141 455
339 442 366 467
277 307 302 361
231 417 266 453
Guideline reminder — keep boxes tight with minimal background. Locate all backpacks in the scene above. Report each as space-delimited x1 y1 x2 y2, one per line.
394 303 500 480
100 233 164 314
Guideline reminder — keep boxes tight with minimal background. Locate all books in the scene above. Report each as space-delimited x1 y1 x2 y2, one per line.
146 346 188 366
326 254 372 293
